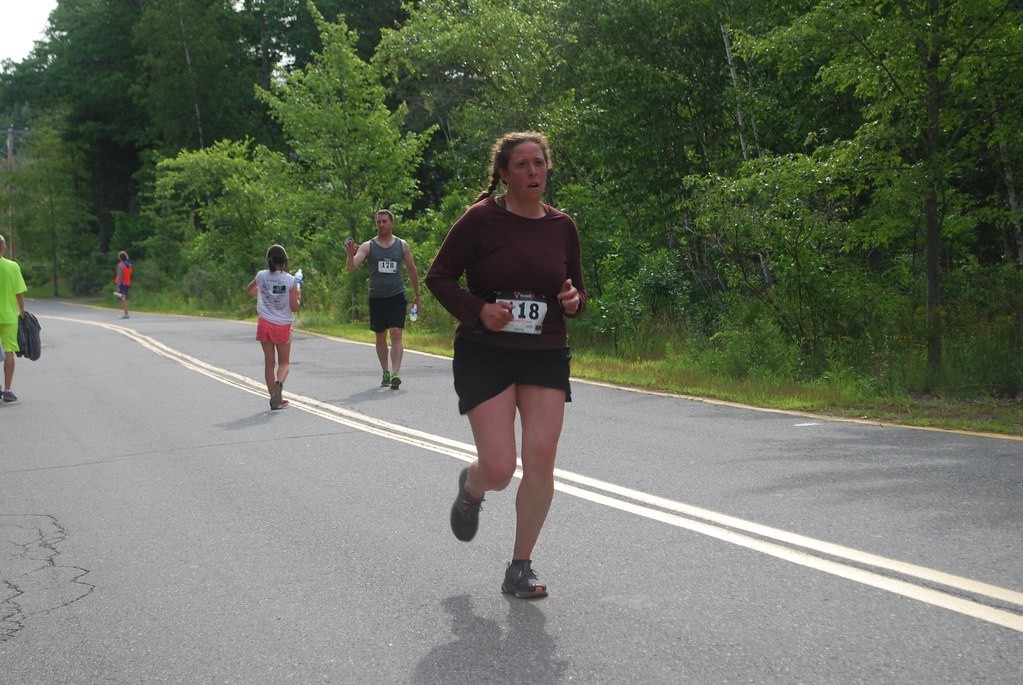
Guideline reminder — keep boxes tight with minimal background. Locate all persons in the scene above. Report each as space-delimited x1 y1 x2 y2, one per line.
0 235 28 401
426 131 588 599
114 251 132 319
247 244 301 411
345 209 421 389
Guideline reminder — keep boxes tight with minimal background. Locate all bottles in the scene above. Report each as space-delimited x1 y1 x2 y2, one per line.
410 304 418 322
113 291 123 298
295 269 304 290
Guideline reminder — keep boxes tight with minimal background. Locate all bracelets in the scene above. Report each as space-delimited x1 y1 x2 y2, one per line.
416 294 420 296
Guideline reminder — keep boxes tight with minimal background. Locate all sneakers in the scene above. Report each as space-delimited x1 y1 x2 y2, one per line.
380 371 391 386
501 559 548 599
451 467 485 542
269 381 283 406
389 371 401 390
271 399 289 410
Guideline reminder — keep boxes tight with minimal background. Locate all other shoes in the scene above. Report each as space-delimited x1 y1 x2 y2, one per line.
2 391 18 401
119 315 129 319
